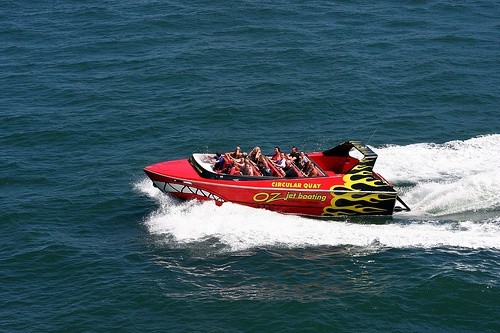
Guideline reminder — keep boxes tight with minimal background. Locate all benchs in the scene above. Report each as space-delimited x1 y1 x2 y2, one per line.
223 152 325 178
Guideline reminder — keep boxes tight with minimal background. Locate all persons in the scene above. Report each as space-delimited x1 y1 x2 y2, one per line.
208 146 270 176
271 145 320 178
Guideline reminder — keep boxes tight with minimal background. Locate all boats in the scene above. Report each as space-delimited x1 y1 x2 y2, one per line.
143 140 411 225
